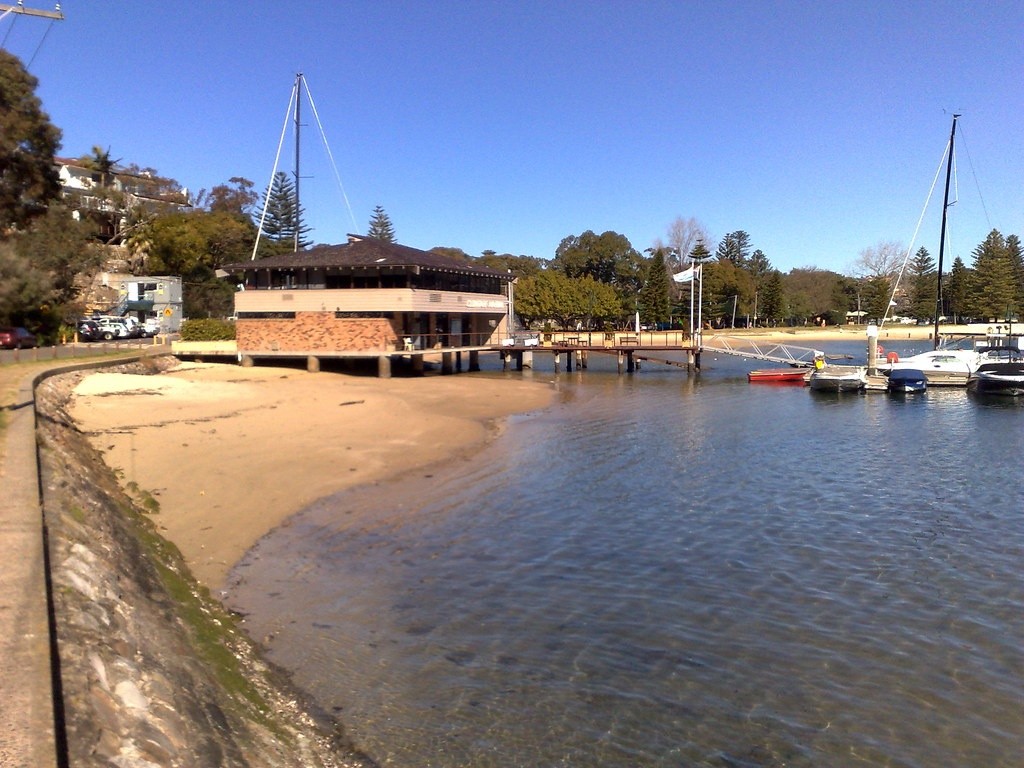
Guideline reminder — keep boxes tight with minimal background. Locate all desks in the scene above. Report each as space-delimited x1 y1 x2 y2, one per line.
567 337 578 346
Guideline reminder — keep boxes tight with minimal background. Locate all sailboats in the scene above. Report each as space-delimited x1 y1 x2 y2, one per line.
876 109 1024 388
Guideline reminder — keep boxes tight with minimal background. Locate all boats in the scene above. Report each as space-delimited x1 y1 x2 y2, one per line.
746 367 812 381
886 369 929 394
810 365 869 391
964 362 1024 396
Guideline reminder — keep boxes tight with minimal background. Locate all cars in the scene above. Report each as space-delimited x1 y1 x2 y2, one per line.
82 314 161 340
74 321 101 340
0 326 37 351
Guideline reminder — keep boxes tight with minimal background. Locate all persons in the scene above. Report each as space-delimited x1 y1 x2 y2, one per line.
817 317 820 325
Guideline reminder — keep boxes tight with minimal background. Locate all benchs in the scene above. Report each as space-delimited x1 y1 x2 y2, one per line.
558 341 567 346
620 337 639 345
578 341 587 346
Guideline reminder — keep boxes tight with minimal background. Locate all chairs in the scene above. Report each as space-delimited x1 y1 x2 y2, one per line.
403 337 414 351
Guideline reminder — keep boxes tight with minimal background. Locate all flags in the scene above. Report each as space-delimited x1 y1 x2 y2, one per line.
672 268 692 282
693 266 700 278
